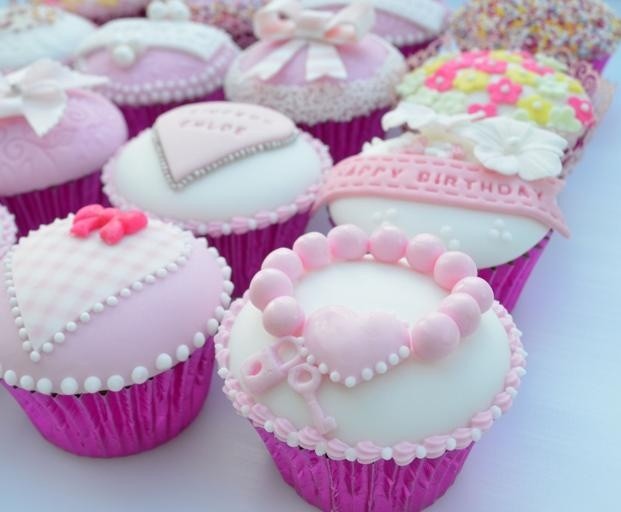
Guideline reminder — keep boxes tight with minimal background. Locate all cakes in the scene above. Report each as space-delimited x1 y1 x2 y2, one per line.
0 0 621 512
